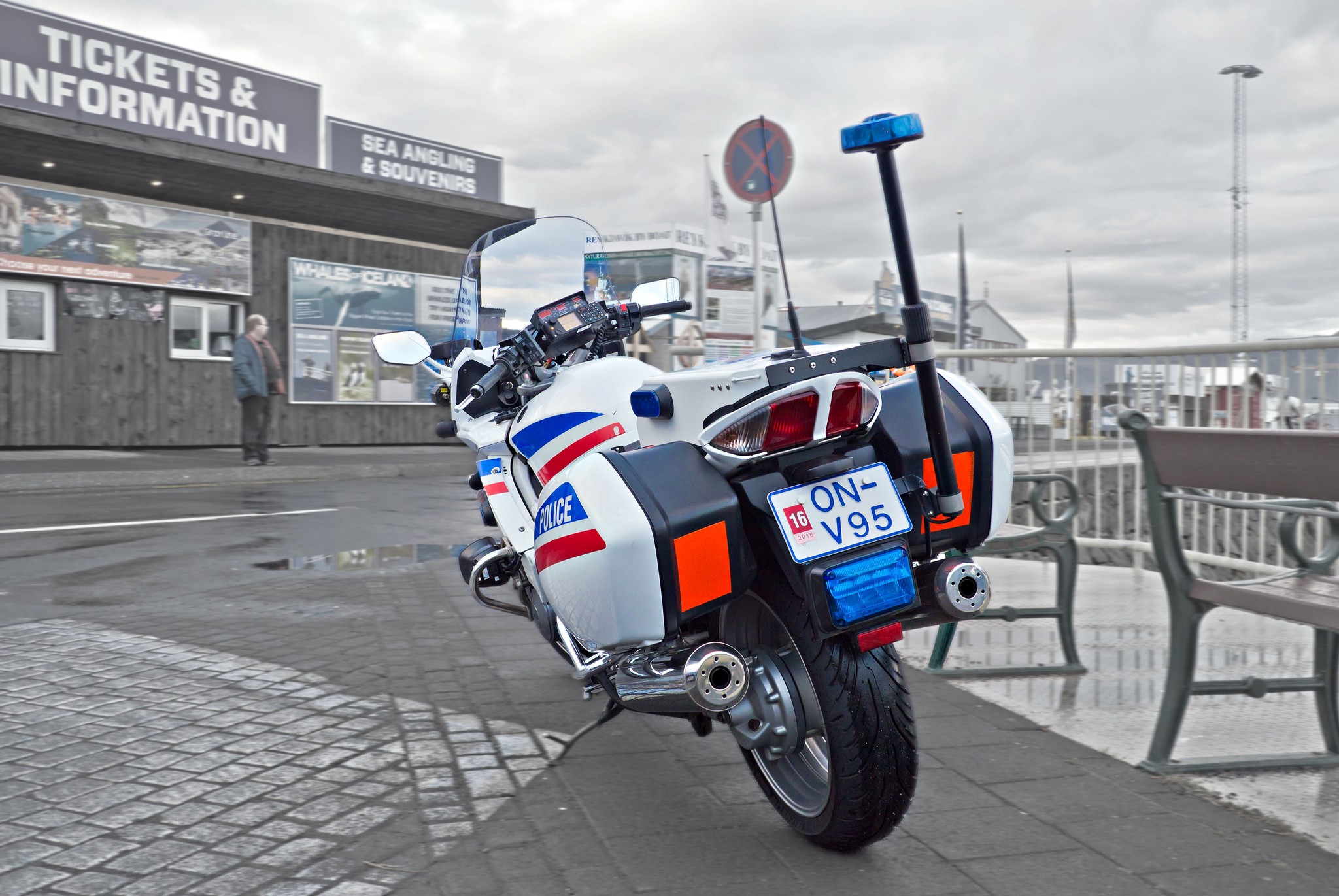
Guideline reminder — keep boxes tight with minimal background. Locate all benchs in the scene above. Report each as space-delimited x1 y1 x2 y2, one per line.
925 472 1091 679
1116 408 1339 775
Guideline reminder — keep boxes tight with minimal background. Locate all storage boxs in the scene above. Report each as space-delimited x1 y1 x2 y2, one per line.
532 441 744 657
873 366 1017 555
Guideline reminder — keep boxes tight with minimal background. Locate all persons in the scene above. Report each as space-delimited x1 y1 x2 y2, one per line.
231 313 286 466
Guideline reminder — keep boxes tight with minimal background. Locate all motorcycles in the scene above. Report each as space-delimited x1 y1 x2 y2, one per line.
370 112 1016 852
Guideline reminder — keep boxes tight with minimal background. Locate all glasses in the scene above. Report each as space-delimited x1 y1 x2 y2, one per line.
258 323 269 327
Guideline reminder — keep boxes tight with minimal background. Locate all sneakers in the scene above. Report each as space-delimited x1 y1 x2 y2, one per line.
244 459 261 466
263 457 278 465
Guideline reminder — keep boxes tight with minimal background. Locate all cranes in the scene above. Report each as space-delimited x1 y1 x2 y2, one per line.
1219 65 1264 344
